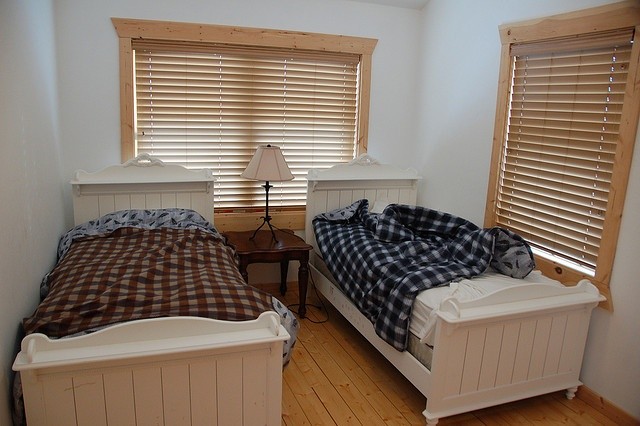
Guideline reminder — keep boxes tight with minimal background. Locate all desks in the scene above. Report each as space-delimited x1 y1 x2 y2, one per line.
223 226 313 317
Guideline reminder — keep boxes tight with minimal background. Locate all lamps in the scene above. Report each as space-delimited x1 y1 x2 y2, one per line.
238 143 296 241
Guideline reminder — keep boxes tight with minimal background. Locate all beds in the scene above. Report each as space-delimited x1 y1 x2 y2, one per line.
302 151 609 424
16 151 297 426
